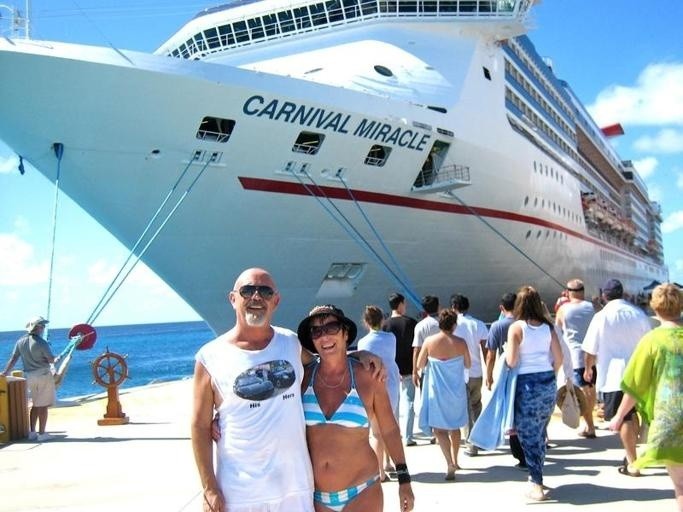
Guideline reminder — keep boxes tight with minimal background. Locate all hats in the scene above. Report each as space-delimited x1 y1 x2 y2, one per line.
26 316 49 334
602 279 623 295
556 385 588 415
297 304 357 354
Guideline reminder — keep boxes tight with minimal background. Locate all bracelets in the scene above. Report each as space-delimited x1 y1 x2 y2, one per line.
393 463 411 484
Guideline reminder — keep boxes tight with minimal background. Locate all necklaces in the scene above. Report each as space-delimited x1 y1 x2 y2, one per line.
315 366 348 389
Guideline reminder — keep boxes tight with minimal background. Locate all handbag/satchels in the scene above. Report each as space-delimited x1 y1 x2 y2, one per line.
561 384 580 429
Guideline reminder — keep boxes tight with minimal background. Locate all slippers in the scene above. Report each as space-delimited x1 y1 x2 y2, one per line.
619 464 639 476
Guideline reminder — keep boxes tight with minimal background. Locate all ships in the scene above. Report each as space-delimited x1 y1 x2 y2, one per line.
0 0 670 353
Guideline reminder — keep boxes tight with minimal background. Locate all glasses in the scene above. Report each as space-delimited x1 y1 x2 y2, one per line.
234 285 277 300
309 320 341 340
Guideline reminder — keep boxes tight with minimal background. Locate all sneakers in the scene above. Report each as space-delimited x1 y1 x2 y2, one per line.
28 432 57 441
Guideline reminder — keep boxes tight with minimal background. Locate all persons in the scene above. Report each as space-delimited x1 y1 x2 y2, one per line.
189 266 388 511
553 278 683 512
352 277 490 480
207 303 418 511
484 285 573 502
0 314 57 441
